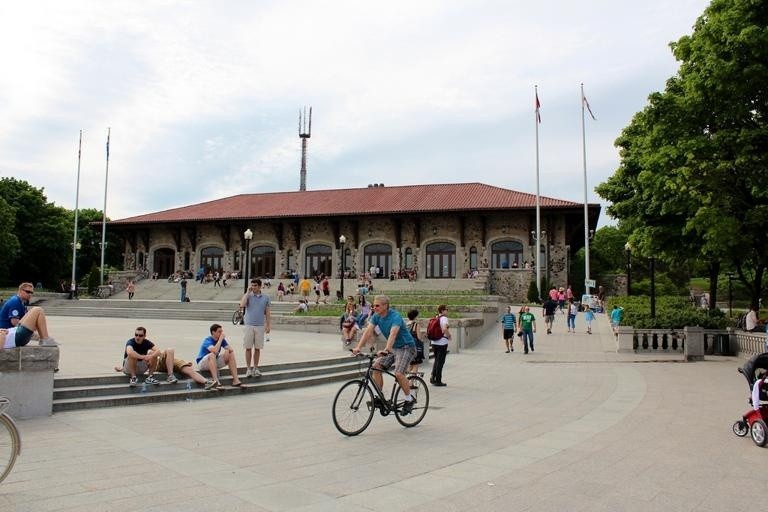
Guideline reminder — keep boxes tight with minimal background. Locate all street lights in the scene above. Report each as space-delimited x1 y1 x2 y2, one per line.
624 241 632 296
242 228 253 324
338 233 345 299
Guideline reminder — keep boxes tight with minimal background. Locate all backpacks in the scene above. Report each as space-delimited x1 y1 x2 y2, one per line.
427 313 444 340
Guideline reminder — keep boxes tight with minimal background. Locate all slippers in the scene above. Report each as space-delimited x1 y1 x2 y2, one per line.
217 383 224 389
232 382 242 386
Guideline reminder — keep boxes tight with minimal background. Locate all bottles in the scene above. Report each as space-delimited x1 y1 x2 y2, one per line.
191 351 196 368
186 378 192 402
266 332 270 341
142 380 146 393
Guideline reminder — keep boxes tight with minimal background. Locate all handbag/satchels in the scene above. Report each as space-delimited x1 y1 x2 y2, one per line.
410 322 425 359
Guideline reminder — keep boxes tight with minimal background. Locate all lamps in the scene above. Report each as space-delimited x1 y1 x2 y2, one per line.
501 224 507 234
306 229 312 236
367 229 372 238
431 226 437 235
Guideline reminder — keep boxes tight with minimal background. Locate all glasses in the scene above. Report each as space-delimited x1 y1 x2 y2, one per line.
135 334 143 337
376 304 379 307
444 309 448 311
23 289 34 294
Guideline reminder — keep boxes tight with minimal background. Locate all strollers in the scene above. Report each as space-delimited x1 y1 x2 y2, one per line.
732 353 768 448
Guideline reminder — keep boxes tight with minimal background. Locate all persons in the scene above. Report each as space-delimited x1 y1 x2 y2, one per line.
0 305 57 349
340 296 451 387
500 305 536 354
199 270 238 288
353 294 417 415
468 267 475 278
357 264 380 294
263 276 272 289
150 272 159 281
123 327 161 387
108 276 114 295
276 263 330 313
389 268 417 282
0 281 33 328
127 280 136 300
241 278 272 376
611 305 624 326
746 304 765 332
196 324 248 389
541 284 604 335
71 280 77 299
169 270 194 282
115 347 217 389
512 261 530 268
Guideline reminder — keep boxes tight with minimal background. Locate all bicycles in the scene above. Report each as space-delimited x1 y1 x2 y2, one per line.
0 397 23 486
332 348 430 437
90 289 106 298
231 305 243 324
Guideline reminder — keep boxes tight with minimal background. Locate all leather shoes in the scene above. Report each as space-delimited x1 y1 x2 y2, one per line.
430 375 436 383
346 339 351 345
401 394 416 416
435 382 446 386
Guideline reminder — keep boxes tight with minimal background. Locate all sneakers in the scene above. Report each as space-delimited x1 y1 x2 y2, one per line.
205 380 216 389
166 374 178 383
40 338 60 346
253 368 262 377
129 375 137 386
145 375 160 384
247 369 252 377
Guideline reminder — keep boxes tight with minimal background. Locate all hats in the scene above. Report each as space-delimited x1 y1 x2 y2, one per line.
559 287 564 291
